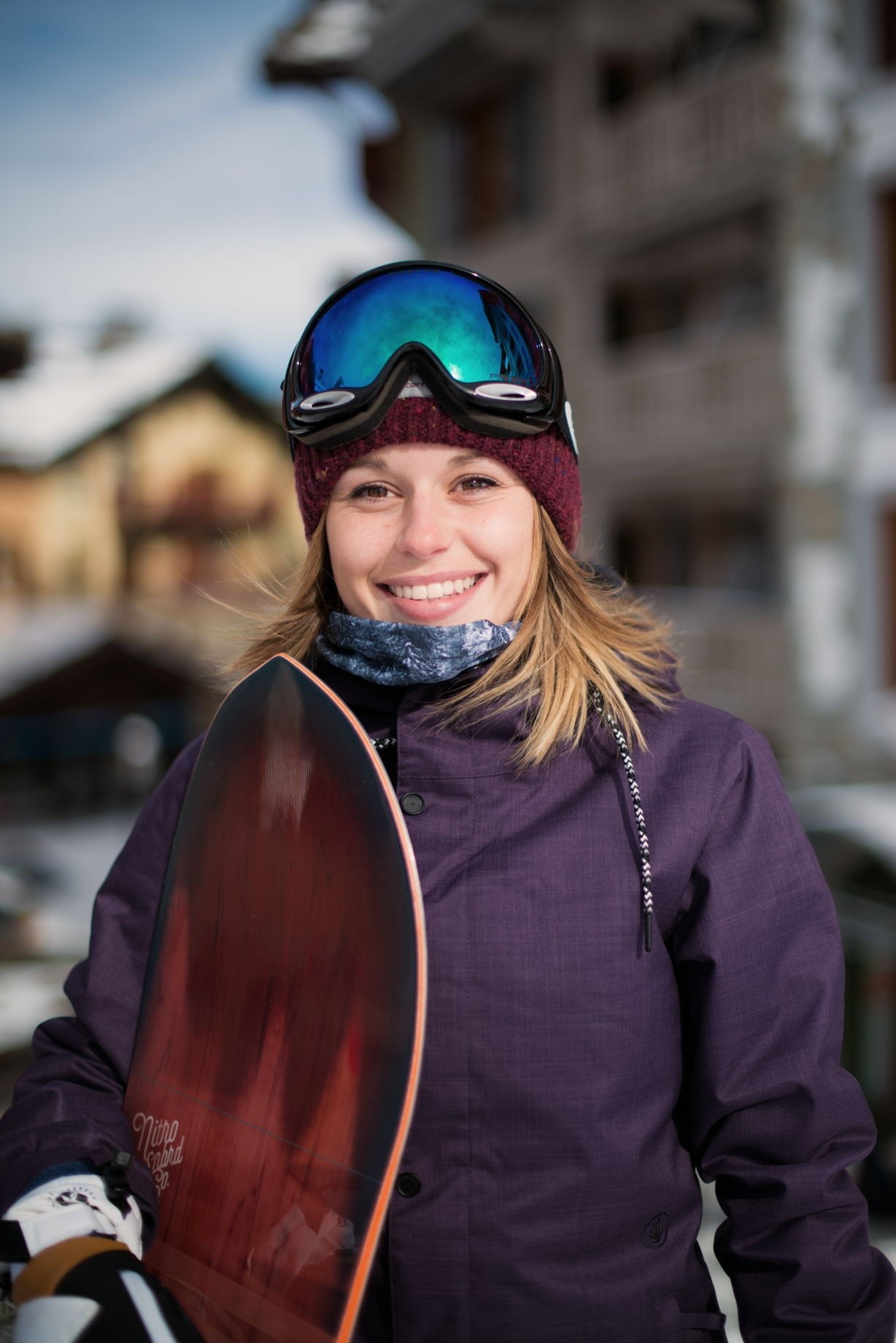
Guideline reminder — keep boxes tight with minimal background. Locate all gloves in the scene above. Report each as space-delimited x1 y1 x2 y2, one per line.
0 1161 206 1343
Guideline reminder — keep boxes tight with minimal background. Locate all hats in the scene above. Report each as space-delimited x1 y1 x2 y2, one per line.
289 368 582 555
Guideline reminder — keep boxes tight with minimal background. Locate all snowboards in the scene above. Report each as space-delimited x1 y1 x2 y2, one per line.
119 650 428 1343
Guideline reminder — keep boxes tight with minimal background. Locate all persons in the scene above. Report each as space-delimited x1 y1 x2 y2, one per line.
1 260 894 1342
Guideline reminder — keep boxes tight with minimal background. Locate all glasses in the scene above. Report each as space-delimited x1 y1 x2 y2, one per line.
282 258 569 447
